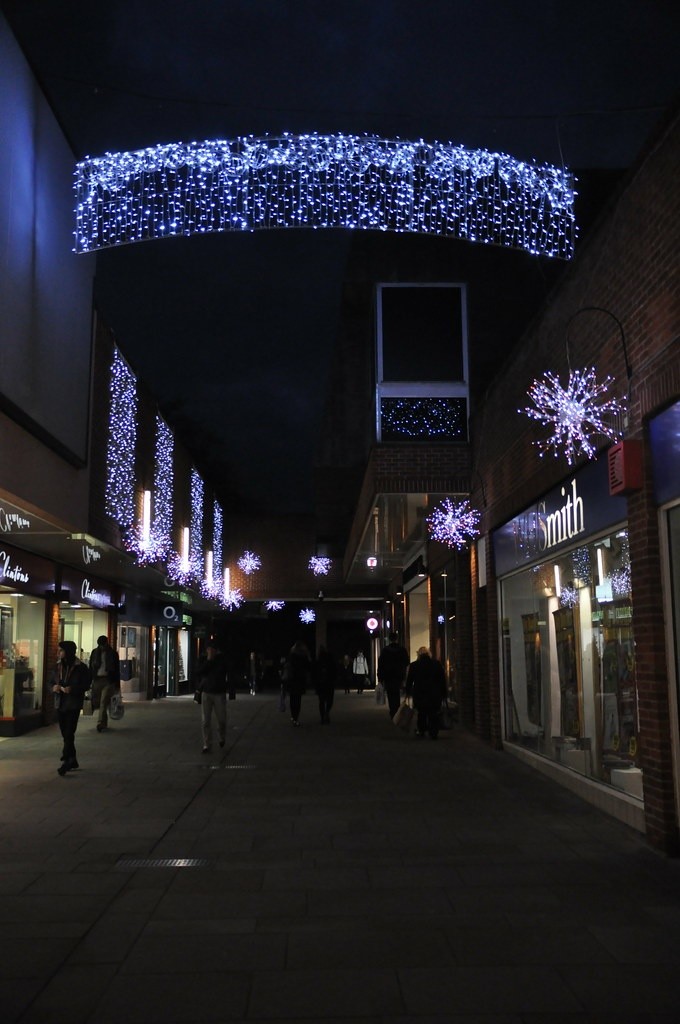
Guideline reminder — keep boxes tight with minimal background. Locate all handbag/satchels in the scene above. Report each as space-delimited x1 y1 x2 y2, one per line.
375 682 386 705
364 678 371 685
83 699 94 716
109 689 124 720
392 694 414 730
436 698 452 730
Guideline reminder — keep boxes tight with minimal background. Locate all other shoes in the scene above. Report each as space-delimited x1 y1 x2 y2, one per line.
97 724 107 732
218 738 225 748
58 757 78 775
290 716 300 727
430 734 437 740
201 746 211 753
414 729 425 737
357 691 363 694
320 712 330 725
249 688 255 695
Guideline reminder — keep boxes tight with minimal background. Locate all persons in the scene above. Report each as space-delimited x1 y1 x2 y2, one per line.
406 647 449 742
377 633 411 720
44 640 92 777
86 635 122 734
244 651 263 697
341 654 354 696
352 650 368 695
193 639 237 755
280 639 313 727
312 644 339 726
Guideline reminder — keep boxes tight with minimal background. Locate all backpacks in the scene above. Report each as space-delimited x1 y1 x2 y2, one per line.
280 654 298 683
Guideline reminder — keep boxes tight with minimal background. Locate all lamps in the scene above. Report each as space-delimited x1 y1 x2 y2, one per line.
423 469 487 553
514 306 633 466
102 523 382 634
